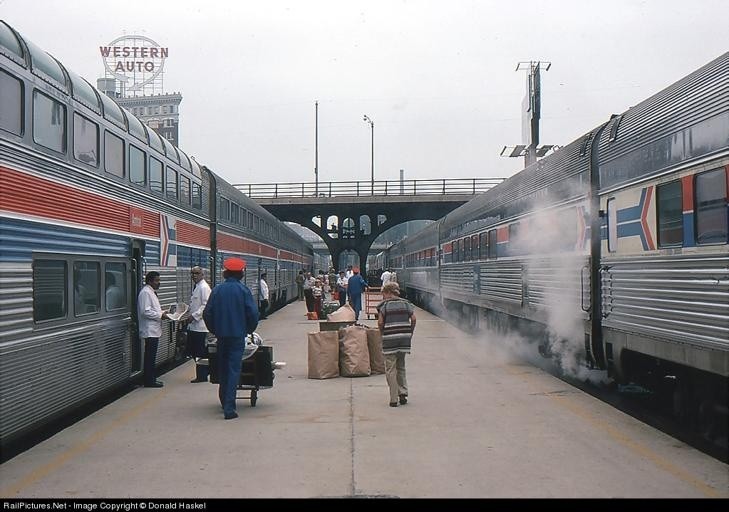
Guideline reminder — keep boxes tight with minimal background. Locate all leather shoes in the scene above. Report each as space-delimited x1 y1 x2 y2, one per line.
191 378 207 383
261 316 268 319
144 381 163 387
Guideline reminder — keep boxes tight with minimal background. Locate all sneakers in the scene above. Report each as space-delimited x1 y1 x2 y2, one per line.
390 399 407 406
225 411 238 419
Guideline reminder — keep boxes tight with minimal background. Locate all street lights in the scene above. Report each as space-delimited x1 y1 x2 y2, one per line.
362 114 375 196
314 97 320 199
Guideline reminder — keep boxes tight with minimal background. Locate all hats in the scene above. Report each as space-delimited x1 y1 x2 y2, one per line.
353 268 359 273
223 257 247 272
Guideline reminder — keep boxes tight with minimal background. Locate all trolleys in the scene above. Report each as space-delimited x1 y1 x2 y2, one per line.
206 329 279 408
364 284 384 322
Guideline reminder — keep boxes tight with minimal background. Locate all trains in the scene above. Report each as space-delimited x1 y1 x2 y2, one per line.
0 19 331 445
367 51 729 455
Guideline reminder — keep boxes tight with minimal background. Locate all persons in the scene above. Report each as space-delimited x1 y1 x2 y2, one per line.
186 265 212 383
258 273 269 320
377 282 416 407
295 265 393 320
201 256 258 419
105 272 124 310
136 271 171 387
62 266 87 313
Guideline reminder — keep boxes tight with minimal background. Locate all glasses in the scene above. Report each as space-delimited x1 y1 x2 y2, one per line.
191 272 202 276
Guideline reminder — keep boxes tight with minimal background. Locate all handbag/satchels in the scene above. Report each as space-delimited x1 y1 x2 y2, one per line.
334 291 340 300
307 312 318 320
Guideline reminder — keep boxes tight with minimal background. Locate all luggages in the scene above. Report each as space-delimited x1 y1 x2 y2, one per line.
320 301 339 320
